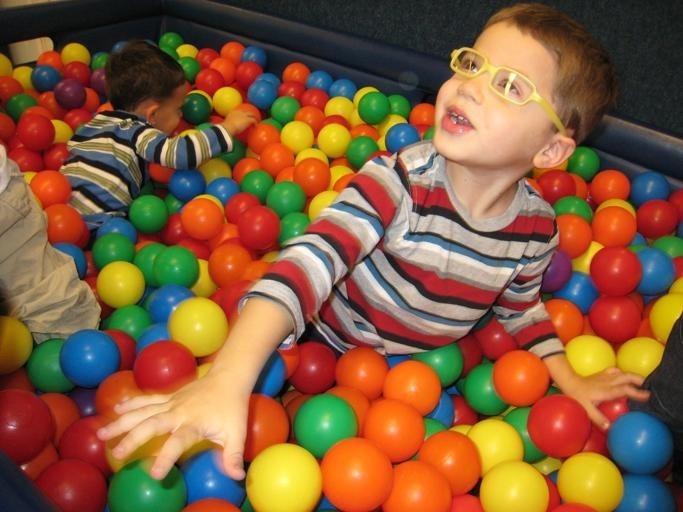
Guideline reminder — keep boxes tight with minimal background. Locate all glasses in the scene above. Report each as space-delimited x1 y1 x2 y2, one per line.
448 47 567 135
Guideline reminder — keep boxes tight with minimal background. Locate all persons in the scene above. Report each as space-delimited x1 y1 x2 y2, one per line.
0 143 103 347
59 43 257 226
625 312 683 485
96 2 652 481
